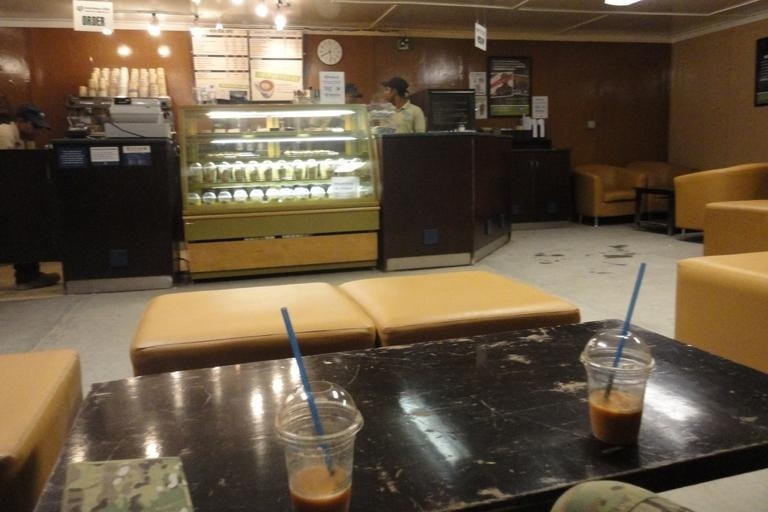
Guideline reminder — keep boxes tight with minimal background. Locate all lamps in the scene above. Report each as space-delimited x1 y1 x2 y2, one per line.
149 14 163 39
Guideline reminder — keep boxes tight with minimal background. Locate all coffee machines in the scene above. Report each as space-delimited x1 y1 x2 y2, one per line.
63 94 176 138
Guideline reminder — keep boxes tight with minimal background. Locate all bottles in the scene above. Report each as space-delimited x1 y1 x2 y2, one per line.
520 114 546 139
200 82 218 105
447 124 466 132
292 86 318 103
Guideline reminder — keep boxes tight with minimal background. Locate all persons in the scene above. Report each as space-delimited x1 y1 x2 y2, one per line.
0 103 61 292
380 75 427 134
308 81 362 154
493 73 512 97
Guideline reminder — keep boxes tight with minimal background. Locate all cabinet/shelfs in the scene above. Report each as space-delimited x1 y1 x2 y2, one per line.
175 104 381 275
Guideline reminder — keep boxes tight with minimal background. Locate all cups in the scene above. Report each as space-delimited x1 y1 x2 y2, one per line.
255 80 275 99
274 380 363 512
578 328 656 449
188 159 336 184
78 66 167 97
186 188 335 205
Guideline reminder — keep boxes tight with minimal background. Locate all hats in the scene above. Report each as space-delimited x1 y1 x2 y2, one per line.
382 77 409 94
344 84 363 96
15 104 53 132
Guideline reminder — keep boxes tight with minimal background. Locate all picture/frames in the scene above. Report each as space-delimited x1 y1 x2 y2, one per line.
486 55 532 118
754 36 768 107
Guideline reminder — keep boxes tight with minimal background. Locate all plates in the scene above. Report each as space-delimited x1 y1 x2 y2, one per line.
304 127 352 136
196 132 244 138
370 127 398 134
371 110 395 117
250 131 298 136
479 126 494 132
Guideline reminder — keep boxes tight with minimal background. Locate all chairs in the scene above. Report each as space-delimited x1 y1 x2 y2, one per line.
574 164 647 227
552 467 768 511
627 159 699 219
672 162 768 242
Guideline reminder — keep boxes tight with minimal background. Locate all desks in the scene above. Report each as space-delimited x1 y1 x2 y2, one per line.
32 320 768 512
633 185 675 237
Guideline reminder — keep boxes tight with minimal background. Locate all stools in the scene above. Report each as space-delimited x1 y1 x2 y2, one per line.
1 349 84 512
337 271 580 346
675 251 768 375
128 282 377 376
704 199 768 256
